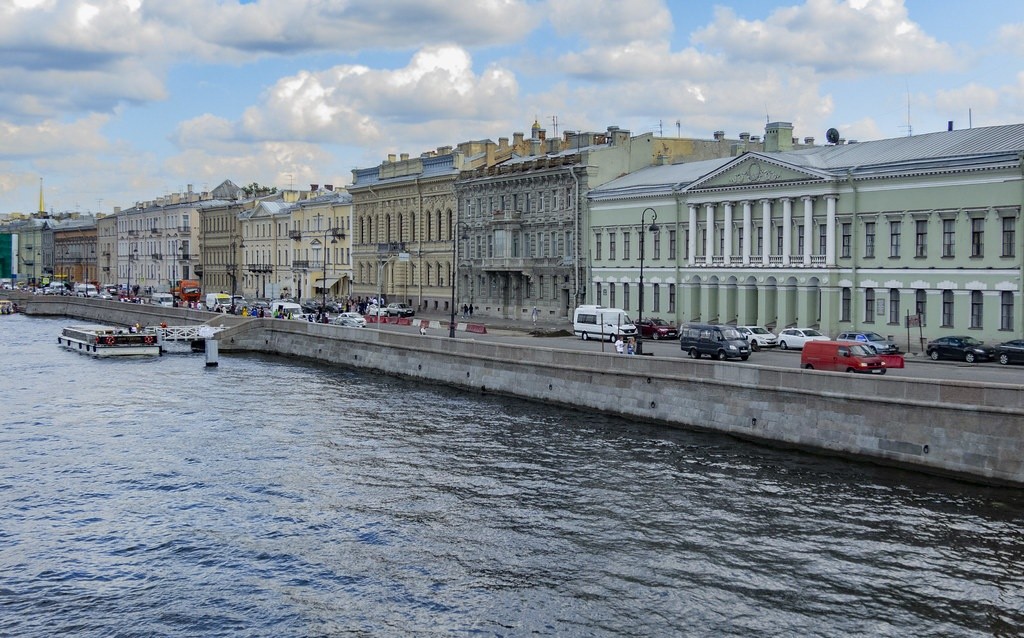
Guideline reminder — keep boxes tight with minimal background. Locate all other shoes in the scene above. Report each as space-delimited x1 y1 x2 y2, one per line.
534 323 536 325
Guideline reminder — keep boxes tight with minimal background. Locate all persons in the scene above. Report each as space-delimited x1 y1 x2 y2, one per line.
627 339 635 354
174 296 201 310
531 306 537 325
215 302 271 318
273 309 329 324
462 304 473 319
614 336 626 353
335 297 384 315
419 324 426 335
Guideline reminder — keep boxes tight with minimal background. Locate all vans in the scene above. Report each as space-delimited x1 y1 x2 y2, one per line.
152 293 173 307
50 282 98 296
272 302 302 320
680 323 751 361
206 294 232 310
573 304 638 343
800 340 886 375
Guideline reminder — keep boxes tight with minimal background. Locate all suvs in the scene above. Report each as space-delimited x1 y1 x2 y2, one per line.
635 317 676 340
387 302 415 318
837 331 899 356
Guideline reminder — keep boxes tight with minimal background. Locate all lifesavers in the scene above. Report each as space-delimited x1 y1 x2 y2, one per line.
144 336 153 343
106 336 115 345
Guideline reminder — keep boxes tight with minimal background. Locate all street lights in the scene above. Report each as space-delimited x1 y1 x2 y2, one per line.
449 221 469 338
128 244 139 299
86 245 96 296
231 236 245 315
173 239 184 307
322 228 338 323
635 208 659 354
11 244 70 296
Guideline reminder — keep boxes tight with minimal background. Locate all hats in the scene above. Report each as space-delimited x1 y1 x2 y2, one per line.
534 306 536 308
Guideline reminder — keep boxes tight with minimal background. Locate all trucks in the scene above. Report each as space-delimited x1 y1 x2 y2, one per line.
170 279 200 303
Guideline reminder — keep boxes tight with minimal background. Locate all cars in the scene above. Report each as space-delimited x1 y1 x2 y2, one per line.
233 296 272 317
302 300 388 328
99 284 139 303
927 335 1024 365
737 325 831 349
3 284 49 295
